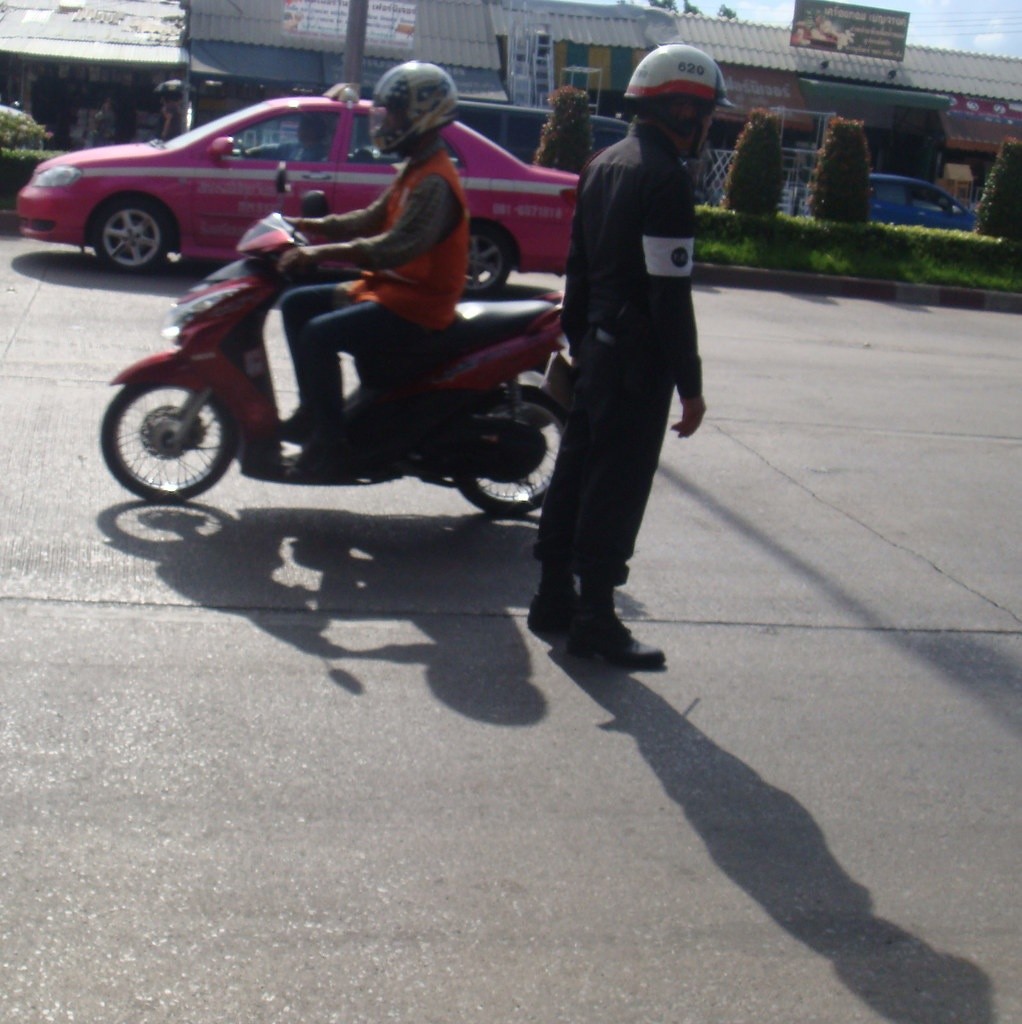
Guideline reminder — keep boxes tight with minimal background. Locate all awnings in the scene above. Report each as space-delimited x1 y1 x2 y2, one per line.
0 0 1022 151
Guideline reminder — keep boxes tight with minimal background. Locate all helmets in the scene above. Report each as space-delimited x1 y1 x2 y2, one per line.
368 59 461 154
623 42 736 109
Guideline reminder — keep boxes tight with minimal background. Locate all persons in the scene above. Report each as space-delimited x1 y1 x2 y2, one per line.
278 62 470 444
246 113 329 161
529 46 733 670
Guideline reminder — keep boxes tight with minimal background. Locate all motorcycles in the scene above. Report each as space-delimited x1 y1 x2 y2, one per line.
103 157 577 518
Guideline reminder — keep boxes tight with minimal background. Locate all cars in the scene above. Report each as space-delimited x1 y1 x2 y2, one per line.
868 172 978 231
17 81 581 300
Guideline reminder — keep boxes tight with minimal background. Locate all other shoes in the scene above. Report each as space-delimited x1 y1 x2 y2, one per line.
564 612 665 670
527 593 572 631
296 439 335 471
272 409 313 439
